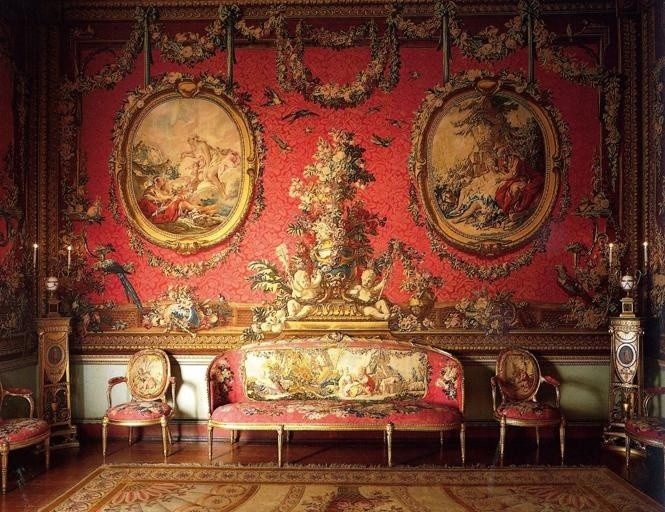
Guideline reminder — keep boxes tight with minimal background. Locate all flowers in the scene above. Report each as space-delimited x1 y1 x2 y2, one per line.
400 248 446 297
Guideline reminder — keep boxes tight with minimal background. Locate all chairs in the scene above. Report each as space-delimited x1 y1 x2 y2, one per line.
624 386 665 474
0 377 51 496
101 348 181 461
489 347 567 467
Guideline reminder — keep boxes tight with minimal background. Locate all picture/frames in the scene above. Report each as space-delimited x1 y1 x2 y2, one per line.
39 1 644 365
640 0 665 372
0 1 40 370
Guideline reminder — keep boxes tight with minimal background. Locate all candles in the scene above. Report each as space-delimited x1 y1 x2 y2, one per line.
66 244 72 268
607 242 613 264
32 241 37 268
643 241 650 264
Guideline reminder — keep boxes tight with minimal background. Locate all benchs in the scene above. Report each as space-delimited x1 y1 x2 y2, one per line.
203 330 469 468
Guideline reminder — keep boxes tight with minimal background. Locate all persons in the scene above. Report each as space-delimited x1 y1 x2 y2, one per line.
449 144 545 223
138 129 246 224
351 268 397 321
284 264 323 320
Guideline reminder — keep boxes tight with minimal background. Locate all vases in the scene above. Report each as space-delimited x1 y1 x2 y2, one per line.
407 291 434 319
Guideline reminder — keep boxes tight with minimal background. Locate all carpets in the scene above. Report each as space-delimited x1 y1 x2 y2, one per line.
36 462 665 512
